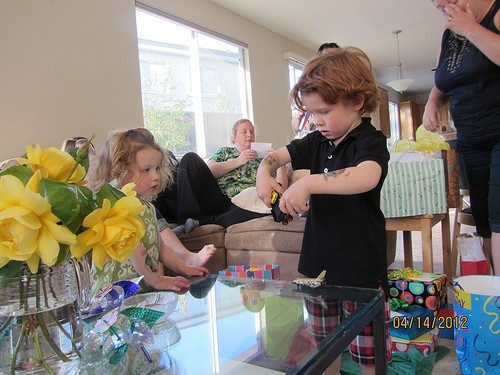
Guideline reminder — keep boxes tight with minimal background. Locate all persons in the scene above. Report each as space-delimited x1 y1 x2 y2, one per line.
256 43 391 375
423 0 500 276
172 119 288 236
88 127 209 300
65 137 216 267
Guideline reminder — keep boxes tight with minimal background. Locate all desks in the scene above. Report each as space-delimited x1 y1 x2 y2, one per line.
83 274 386 375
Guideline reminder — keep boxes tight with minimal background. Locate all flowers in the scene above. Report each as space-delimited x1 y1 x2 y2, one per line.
0 134 147 375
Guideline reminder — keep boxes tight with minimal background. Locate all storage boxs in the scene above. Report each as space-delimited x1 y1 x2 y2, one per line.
388 272 449 357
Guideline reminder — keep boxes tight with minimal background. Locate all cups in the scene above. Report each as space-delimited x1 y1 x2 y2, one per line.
452 275 499 375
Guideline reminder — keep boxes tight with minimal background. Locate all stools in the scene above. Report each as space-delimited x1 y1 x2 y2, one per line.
450 189 476 276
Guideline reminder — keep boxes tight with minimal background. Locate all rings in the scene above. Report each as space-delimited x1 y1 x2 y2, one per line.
448 16 455 22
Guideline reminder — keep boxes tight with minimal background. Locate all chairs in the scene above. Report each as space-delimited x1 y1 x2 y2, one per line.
386 150 451 273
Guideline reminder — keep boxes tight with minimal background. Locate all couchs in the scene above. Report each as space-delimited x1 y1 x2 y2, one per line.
168 169 311 272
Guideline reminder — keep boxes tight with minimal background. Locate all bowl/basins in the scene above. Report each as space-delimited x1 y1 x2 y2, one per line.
143 317 181 349
122 292 179 324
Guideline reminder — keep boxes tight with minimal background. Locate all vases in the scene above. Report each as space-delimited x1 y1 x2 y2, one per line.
0 258 84 375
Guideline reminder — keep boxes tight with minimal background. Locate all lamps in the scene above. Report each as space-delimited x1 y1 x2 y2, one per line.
387 30 414 94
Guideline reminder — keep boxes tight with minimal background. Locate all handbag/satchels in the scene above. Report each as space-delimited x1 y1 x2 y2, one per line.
456 231 491 276
380 135 447 218
